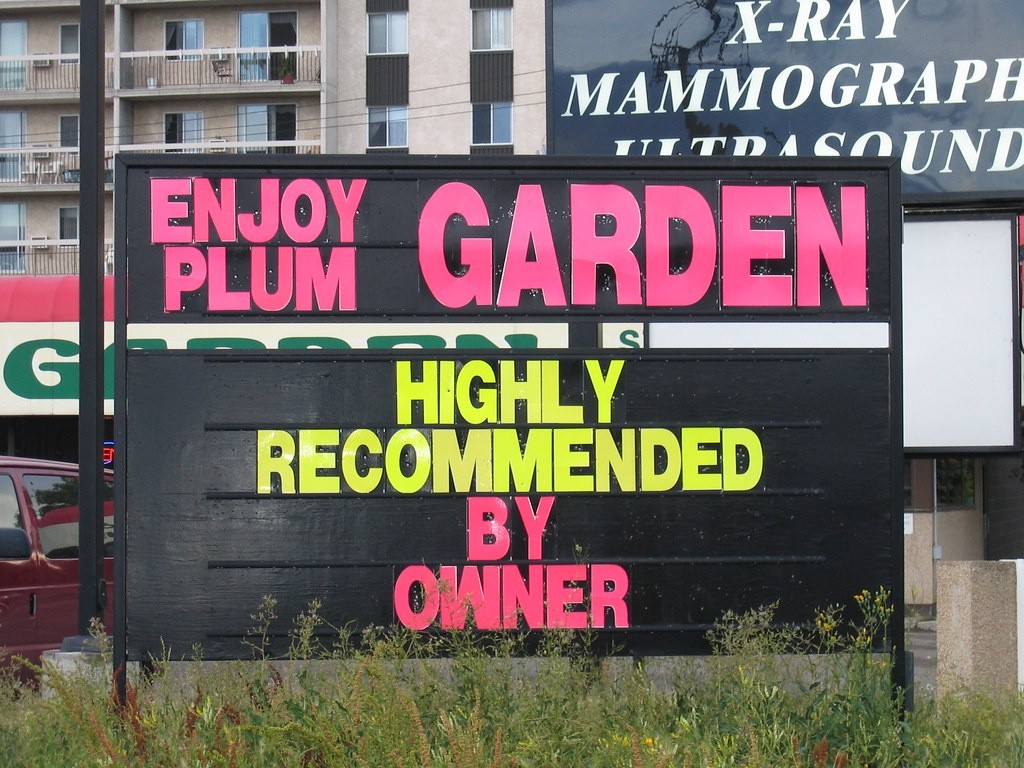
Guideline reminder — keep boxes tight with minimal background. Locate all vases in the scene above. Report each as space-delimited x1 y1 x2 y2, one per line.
147 77 158 89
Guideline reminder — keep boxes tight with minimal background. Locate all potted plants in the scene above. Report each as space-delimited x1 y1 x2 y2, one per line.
280 58 295 84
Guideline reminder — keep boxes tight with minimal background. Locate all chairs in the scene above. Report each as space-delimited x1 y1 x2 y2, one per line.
22 162 41 185
210 60 234 84
40 161 64 185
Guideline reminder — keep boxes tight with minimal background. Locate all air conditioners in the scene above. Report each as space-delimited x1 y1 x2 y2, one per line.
208 139 229 152
32 144 52 158
32 52 53 68
30 236 49 249
208 45 230 60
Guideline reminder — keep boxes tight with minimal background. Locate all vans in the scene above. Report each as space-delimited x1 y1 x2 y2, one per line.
0 454 117 685
